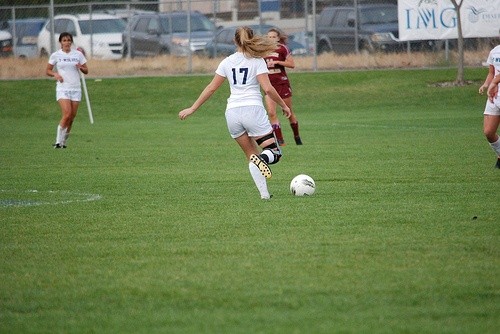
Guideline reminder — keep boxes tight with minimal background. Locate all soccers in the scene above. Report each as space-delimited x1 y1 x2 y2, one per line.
290 175 316 197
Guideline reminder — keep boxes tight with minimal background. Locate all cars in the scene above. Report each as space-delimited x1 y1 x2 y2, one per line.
203 24 308 57
0 30 13 58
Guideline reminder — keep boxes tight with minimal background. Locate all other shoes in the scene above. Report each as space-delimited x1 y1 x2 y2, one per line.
294 136 302 145
495 158 500 169
278 140 285 146
250 153 272 179
63 145 66 148
52 143 61 148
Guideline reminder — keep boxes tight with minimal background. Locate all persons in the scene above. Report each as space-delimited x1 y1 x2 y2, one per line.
479 45 500 168
264 29 302 146
46 32 88 148
488 74 500 102
179 26 291 198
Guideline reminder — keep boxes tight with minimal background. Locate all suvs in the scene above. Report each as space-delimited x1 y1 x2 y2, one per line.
36 13 128 61
121 10 224 58
311 3 444 57
